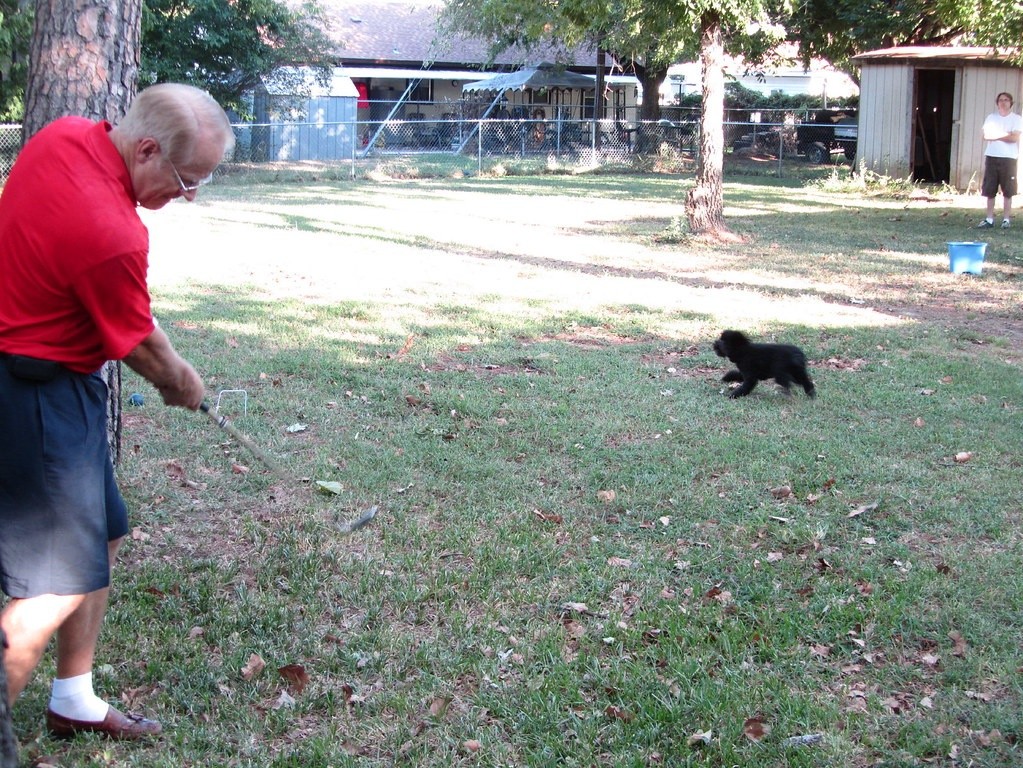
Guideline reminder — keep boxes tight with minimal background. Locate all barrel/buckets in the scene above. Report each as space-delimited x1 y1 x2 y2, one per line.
947 241 987 276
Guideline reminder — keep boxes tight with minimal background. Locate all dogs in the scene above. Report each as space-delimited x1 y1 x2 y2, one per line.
715 331 817 398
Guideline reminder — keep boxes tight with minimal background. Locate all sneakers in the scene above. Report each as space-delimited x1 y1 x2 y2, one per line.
1000 221 1009 229
977 220 994 228
46 704 162 742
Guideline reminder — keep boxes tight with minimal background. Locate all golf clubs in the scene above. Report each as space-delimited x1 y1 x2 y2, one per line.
199 400 379 534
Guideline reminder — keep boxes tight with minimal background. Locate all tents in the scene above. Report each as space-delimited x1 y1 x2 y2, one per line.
462 60 607 158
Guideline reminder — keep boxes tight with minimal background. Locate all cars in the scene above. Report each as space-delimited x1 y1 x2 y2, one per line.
797 109 858 164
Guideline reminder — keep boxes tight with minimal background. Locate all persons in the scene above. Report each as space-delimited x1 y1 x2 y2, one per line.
977 93 1020 229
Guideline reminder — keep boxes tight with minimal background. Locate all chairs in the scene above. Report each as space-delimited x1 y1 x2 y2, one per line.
411 113 692 156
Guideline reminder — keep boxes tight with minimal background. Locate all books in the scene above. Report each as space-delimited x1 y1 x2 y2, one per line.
0 83 235 740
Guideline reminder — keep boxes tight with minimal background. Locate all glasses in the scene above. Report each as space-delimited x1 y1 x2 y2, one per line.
167 158 214 191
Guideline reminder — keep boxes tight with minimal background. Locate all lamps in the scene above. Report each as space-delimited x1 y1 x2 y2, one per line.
501 96 509 109
